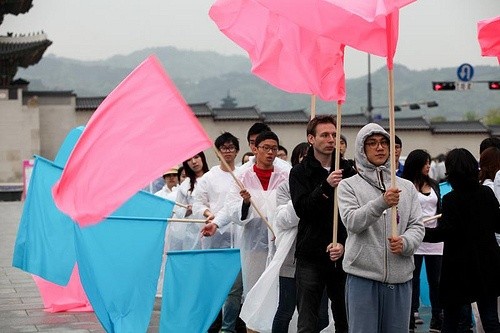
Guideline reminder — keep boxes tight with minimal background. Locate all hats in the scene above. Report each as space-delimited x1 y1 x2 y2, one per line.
163 165 179 174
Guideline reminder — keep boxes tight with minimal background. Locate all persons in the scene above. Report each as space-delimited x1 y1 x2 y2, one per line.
156 115 500 333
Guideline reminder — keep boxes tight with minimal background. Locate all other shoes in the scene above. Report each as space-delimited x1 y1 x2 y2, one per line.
429 314 443 332
414 313 423 323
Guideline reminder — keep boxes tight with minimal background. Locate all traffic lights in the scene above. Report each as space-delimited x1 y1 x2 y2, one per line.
432 81 456 91
488 81 500 90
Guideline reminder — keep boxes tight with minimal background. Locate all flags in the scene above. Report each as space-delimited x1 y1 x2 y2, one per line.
12 122 175 333
208 0 346 105
476 16 500 65
254 0 417 70
52 53 213 228
158 248 241 333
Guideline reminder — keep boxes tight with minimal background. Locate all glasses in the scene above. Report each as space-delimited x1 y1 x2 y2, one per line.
220 145 237 152
365 140 390 149
258 145 279 153
